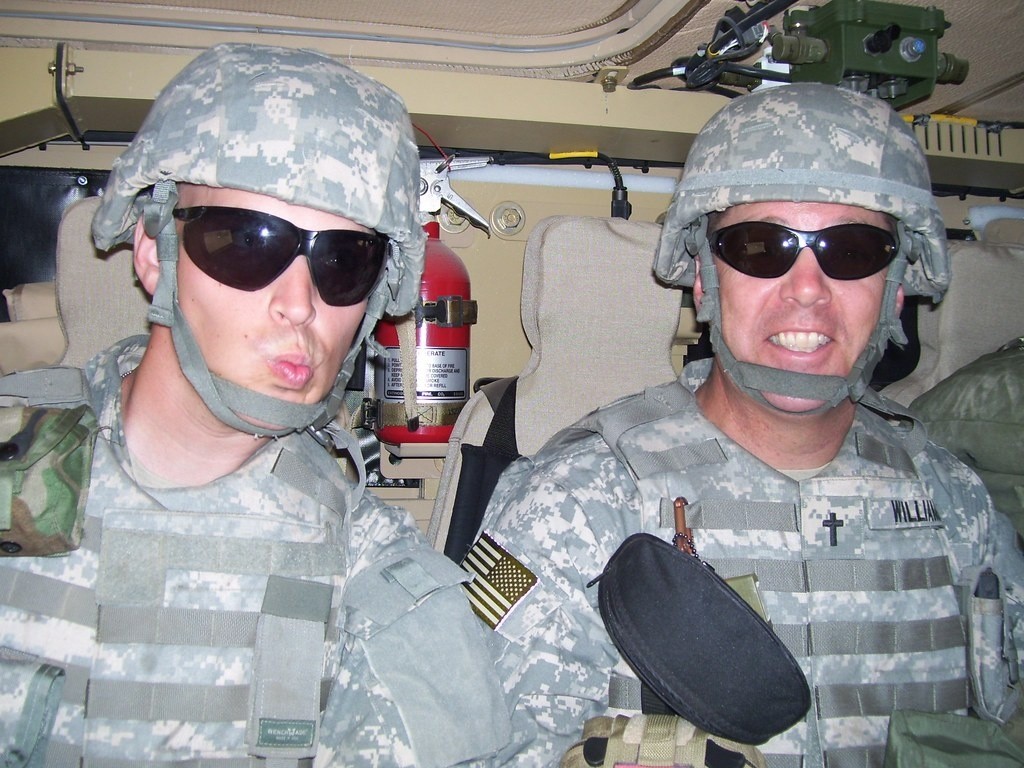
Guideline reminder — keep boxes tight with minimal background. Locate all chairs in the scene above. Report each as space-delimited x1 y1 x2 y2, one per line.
877 240 1024 409
427 217 683 564
53 197 151 368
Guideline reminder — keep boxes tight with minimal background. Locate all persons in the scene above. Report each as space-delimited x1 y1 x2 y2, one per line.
1 44 512 768
461 83 1024 767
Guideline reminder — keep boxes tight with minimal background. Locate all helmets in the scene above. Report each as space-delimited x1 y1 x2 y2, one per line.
655 81 953 303
93 40 424 314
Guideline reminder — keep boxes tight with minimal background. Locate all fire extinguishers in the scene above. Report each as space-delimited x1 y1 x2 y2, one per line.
371 156 494 460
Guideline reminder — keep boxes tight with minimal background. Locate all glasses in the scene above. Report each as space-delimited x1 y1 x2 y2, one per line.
171 206 395 308
709 221 899 282
